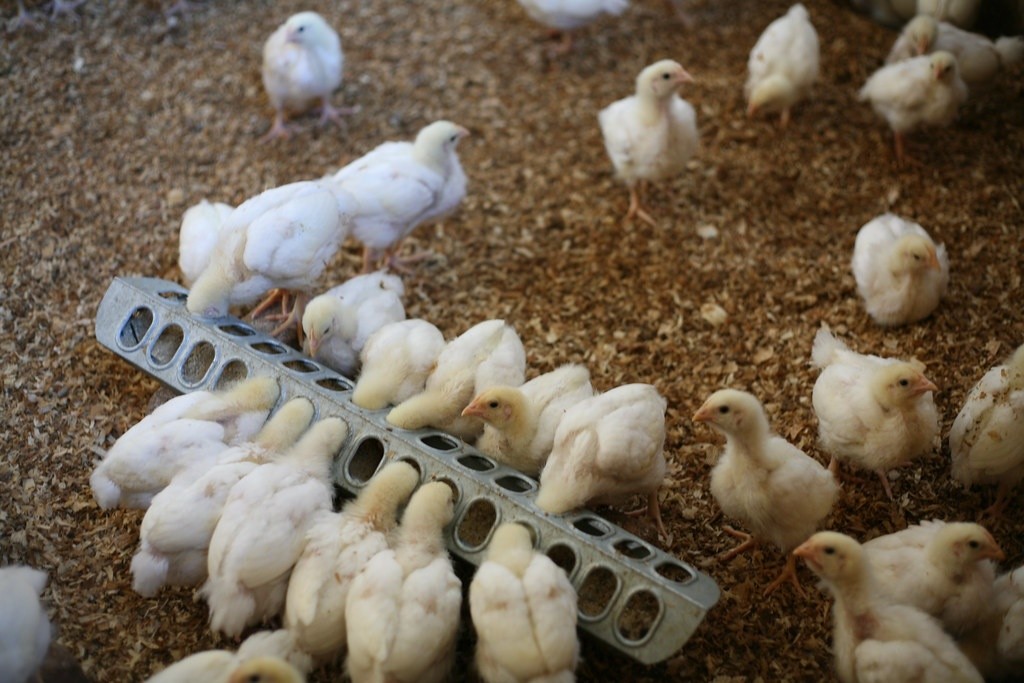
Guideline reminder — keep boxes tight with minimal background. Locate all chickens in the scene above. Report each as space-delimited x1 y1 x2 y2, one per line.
806 326 941 502
742 5 818 128
352 318 444 414
0 372 463 683
384 319 526 438
264 13 363 143
690 388 843 598
467 520 580 682
518 0 629 54
296 267 404 369
460 358 595 470
177 181 346 346
949 343 1024 524
854 50 965 172
595 61 699 228
535 384 672 540
887 17 1024 105
852 213 948 324
329 118 473 275
787 517 1023 682
